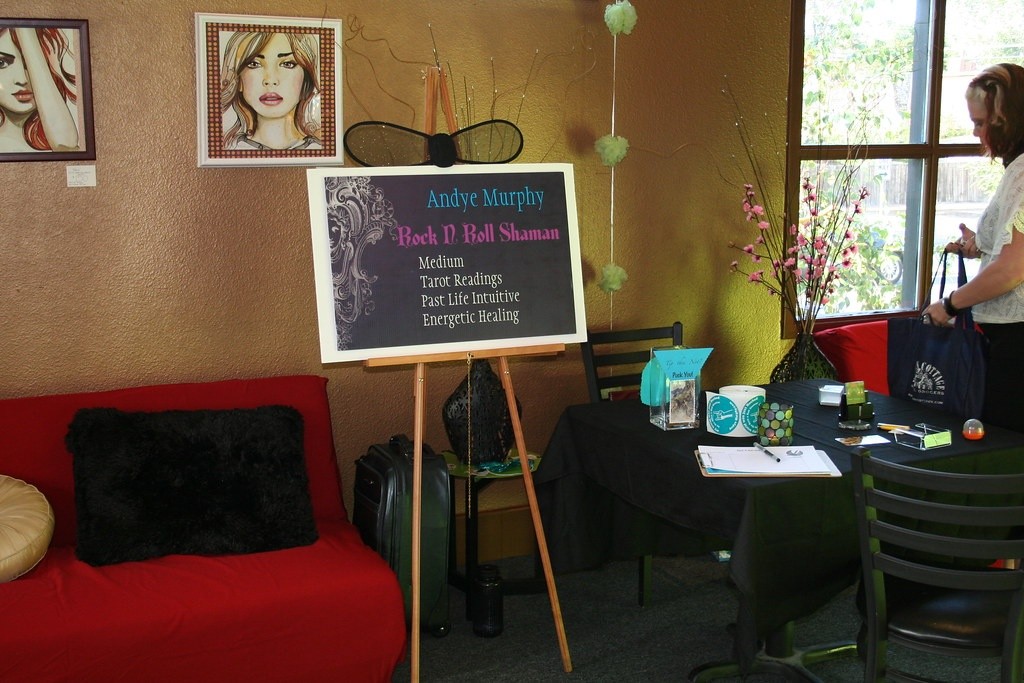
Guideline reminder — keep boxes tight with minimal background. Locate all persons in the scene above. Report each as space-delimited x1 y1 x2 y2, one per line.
921 63 1024 430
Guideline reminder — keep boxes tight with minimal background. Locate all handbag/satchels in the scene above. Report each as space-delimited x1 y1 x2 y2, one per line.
887 243 989 419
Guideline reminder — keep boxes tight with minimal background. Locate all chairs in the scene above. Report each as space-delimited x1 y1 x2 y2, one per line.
851 446 1024 683
582 321 683 606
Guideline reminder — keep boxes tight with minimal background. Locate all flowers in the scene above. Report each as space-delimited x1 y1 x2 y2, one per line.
721 74 880 333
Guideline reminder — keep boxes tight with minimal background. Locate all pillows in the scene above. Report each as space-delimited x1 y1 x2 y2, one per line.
65 405 319 568
0 475 55 583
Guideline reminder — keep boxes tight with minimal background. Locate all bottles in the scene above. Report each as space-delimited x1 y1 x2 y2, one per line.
472 566 504 638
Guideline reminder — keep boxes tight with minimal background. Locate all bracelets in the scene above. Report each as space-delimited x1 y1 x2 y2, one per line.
942 290 961 317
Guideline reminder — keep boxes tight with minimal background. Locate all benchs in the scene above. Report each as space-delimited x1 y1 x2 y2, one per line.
0 376 408 683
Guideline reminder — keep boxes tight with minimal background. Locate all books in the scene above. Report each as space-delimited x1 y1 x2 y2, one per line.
698 445 832 474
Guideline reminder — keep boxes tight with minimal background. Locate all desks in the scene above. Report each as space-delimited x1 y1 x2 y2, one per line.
533 377 1024 683
443 448 548 622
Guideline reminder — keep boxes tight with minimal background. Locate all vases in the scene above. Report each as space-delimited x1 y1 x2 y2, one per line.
442 358 524 464
770 335 842 382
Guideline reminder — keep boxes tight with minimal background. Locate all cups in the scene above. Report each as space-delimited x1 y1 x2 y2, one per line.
649 345 700 431
758 401 794 447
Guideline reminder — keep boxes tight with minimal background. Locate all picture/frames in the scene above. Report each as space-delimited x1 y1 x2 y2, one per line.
195 12 344 168
0 17 96 162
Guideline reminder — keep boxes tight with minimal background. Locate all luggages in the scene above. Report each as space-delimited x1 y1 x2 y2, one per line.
352 433 451 638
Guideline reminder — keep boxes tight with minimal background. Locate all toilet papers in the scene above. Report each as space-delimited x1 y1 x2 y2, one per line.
705 385 767 439
